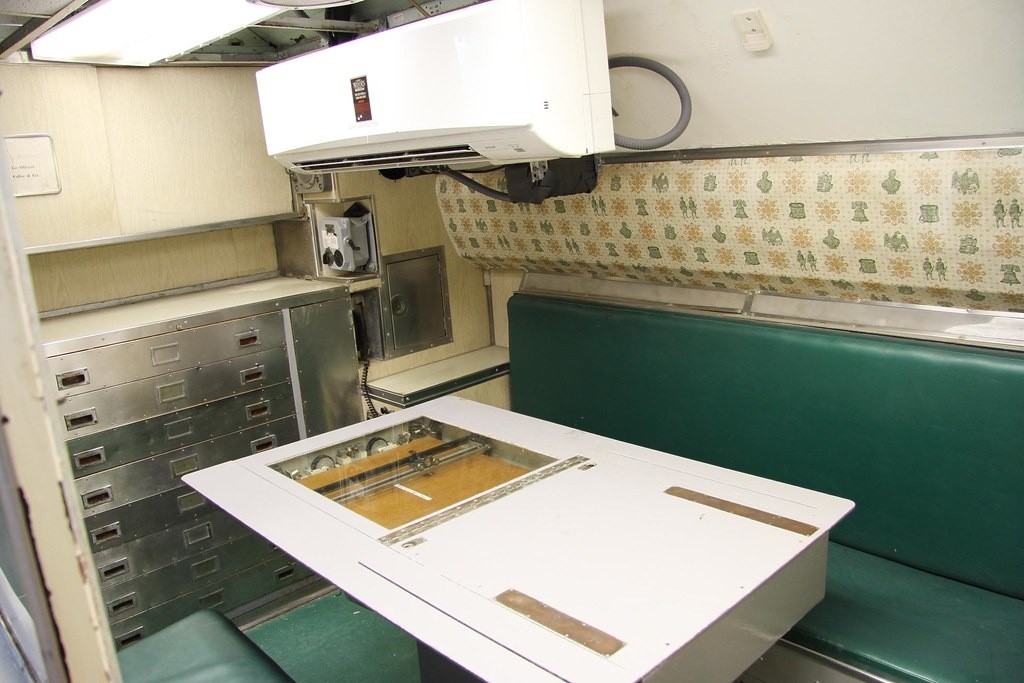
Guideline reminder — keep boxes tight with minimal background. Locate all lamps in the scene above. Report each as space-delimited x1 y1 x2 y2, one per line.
30 0 287 67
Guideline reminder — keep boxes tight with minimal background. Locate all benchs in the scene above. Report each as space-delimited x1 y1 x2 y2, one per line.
504 289 1024 683
118 610 298 683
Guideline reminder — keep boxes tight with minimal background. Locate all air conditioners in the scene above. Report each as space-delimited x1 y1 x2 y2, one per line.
254 0 616 176
